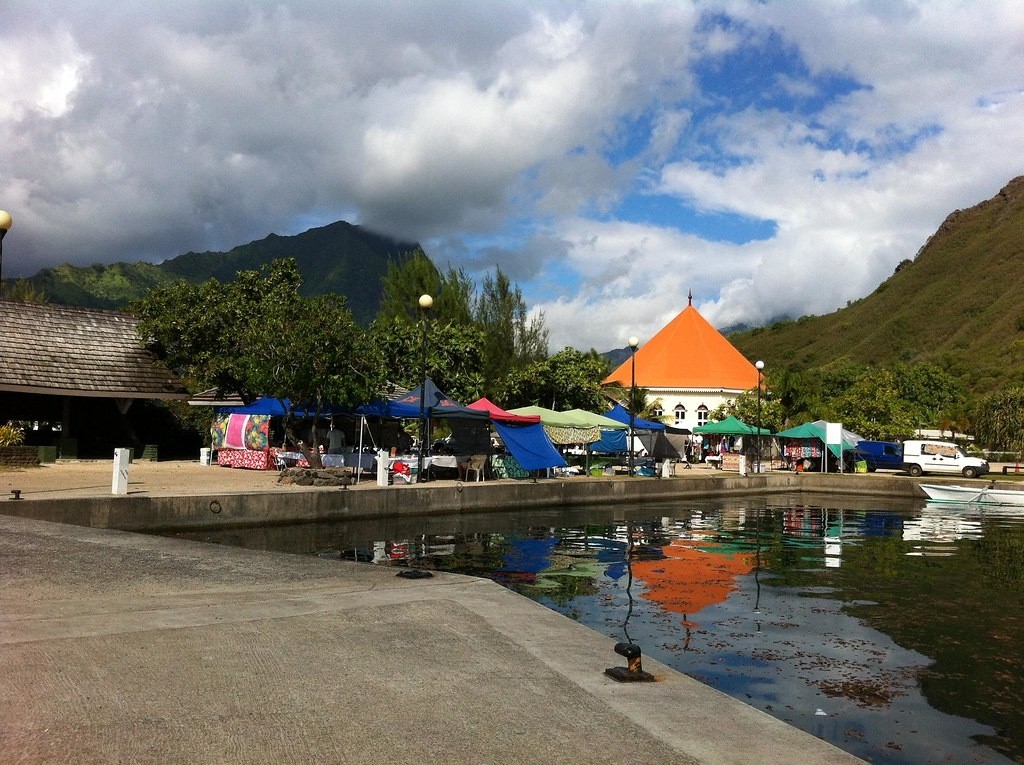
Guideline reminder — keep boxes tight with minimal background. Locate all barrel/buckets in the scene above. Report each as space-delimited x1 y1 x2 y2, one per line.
200 448 211 466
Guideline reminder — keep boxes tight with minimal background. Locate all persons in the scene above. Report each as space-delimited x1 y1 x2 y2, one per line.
693 440 700 464
398 427 414 454
684 437 692 463
327 421 346 453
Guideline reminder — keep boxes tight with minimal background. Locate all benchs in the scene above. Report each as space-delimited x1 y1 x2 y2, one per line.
1002 466 1024 475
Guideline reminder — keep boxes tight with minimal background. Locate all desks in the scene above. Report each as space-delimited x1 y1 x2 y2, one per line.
278 452 531 487
705 455 722 469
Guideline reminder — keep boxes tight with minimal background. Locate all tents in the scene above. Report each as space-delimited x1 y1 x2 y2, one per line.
601 404 666 468
466 397 567 483
772 419 866 472
396 378 490 469
562 409 631 475
692 415 771 466
221 390 430 482
508 405 594 476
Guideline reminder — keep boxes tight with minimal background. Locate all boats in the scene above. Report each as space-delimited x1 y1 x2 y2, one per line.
918 483 1024 506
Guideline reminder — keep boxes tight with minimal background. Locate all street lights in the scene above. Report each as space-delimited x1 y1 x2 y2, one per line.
0 211 12 285
629 336 639 478
416 294 433 483
756 360 764 474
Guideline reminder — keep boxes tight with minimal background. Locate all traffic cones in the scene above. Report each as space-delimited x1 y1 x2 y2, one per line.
1015 462 1020 472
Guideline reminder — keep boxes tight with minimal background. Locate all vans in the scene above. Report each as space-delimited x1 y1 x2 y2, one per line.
903 440 990 478
855 441 903 472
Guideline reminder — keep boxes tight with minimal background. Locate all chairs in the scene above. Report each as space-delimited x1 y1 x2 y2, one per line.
668 458 678 476
464 455 487 483
